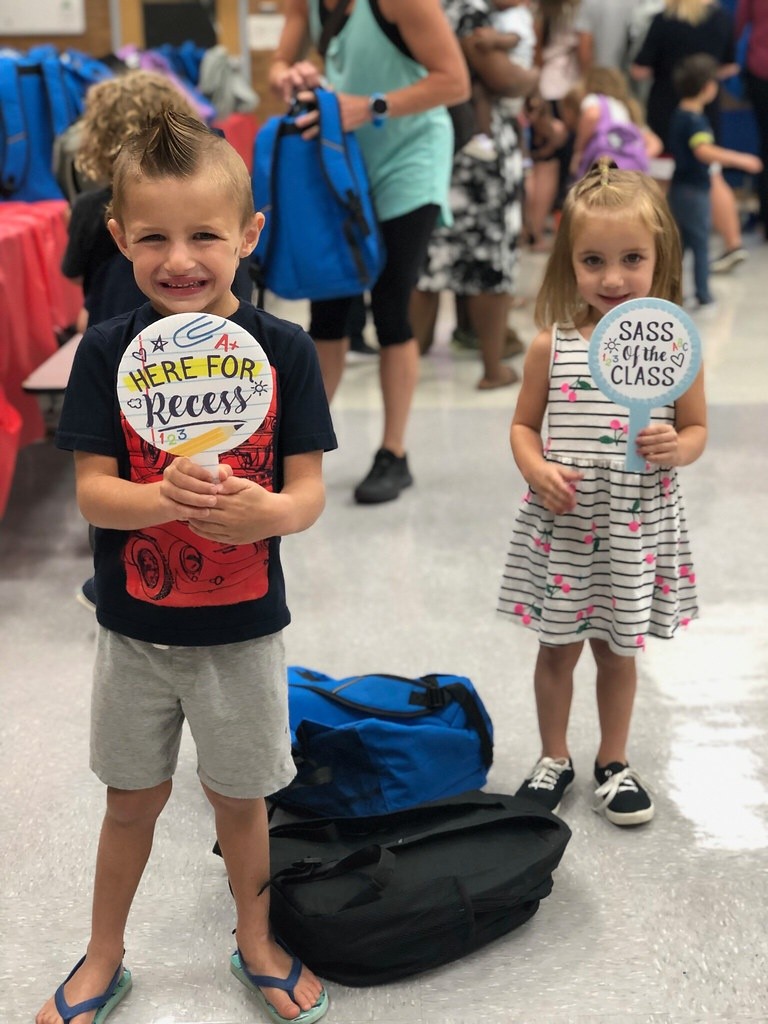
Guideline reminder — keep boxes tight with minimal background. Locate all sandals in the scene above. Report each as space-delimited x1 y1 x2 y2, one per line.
54 953 132 1024
228 937 328 1024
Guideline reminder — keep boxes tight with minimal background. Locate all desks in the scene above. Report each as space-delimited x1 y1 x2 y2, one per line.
0 112 257 518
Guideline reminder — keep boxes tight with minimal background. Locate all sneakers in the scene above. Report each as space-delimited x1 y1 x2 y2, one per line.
355 447 413 503
514 757 575 816
592 755 654 825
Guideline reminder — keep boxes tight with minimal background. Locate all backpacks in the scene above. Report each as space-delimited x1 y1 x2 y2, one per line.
578 94 652 179
246 86 385 310
265 664 495 820
0 40 256 205
210 787 572 989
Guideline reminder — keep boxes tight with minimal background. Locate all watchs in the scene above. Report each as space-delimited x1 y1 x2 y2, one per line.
369 92 389 126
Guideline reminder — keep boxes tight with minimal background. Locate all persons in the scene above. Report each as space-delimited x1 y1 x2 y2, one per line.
31 102 340 1024
412 1 768 391
60 71 192 328
498 165 709 825
268 0 472 503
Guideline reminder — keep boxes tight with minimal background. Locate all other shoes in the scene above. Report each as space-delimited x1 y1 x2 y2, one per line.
708 247 748 274
